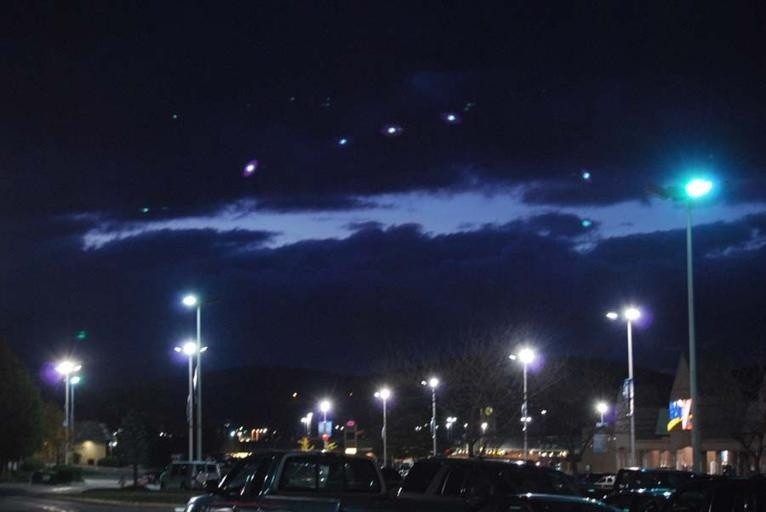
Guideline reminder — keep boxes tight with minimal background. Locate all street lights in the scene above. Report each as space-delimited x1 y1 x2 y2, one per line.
624 308 642 460
181 295 203 462
680 173 721 474
516 347 535 454
377 386 393 468
429 377 441 458
320 400 331 433
54 360 84 467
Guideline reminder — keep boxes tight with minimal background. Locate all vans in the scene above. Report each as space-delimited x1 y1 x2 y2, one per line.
160 461 221 490
